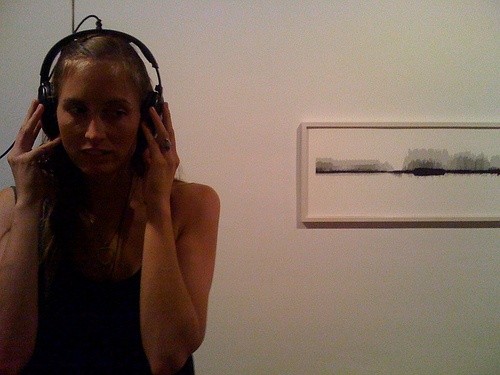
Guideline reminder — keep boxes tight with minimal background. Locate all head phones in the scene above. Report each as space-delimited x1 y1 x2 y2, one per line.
39 29 164 147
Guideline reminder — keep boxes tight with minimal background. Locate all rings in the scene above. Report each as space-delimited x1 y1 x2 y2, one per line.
159 138 171 153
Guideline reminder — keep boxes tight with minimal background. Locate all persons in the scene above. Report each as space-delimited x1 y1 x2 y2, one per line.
0 16 220 375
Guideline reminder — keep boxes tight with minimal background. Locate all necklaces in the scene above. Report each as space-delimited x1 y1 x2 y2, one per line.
65 172 133 268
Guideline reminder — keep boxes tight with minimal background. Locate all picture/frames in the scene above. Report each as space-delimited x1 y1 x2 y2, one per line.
299 121 500 222
